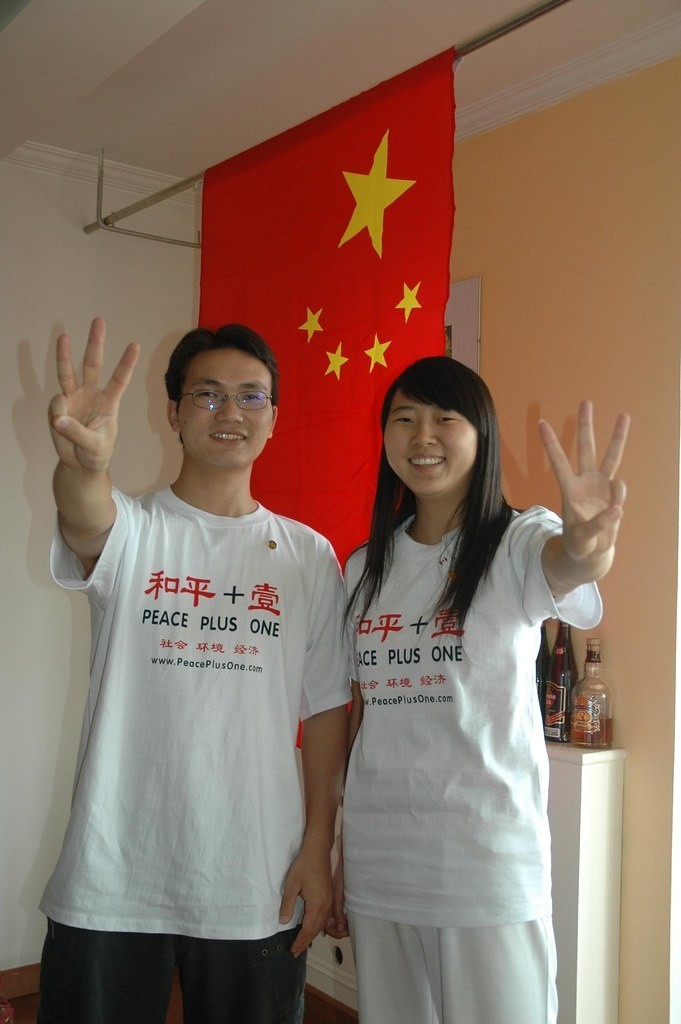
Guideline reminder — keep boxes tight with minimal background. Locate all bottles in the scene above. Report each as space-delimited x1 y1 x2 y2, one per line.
571 638 613 748
543 619 579 742
536 621 550 741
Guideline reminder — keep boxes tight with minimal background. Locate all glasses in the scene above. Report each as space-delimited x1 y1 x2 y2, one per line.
179 387 273 410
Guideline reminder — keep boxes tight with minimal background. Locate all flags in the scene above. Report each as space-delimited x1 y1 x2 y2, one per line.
196 49 459 754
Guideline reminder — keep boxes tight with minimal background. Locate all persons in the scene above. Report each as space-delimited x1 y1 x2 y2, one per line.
31 316 351 1024
323 353 633 1023
